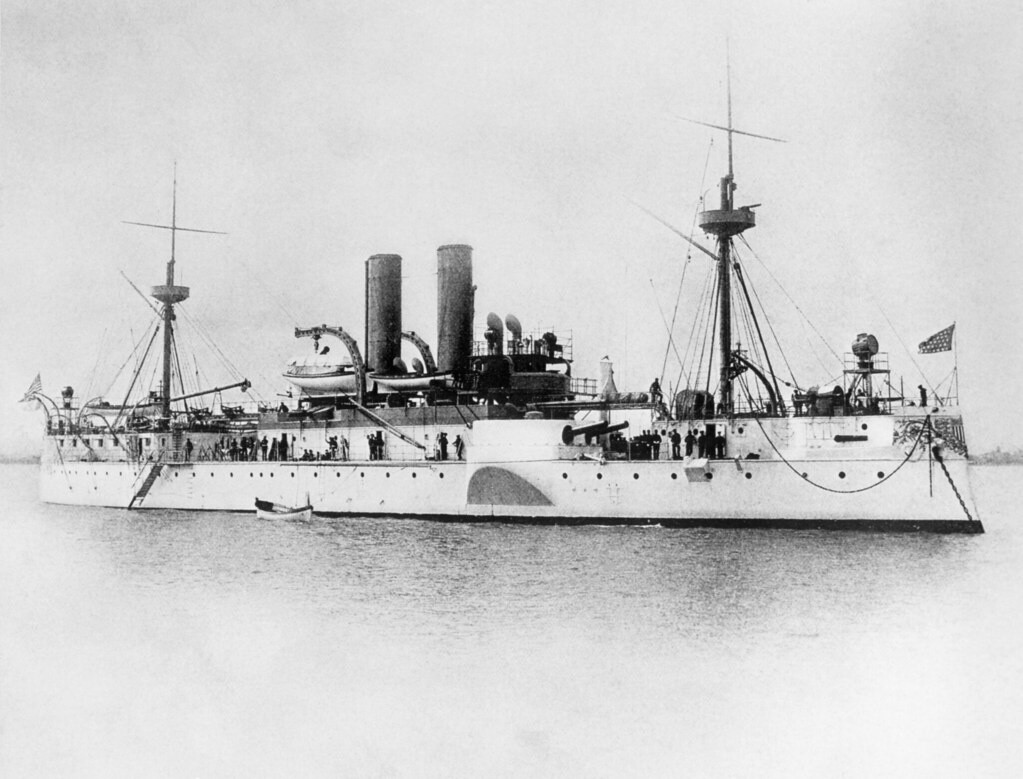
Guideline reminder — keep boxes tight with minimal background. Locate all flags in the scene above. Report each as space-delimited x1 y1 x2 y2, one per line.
918 324 954 353
19 372 41 401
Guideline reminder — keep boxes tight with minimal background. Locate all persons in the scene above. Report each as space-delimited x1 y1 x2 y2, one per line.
649 378 663 404
215 436 289 461
434 432 449 458
917 385 929 406
278 402 288 414
452 435 465 460
299 430 386 462
855 389 865 407
182 438 194 462
793 389 803 417
606 431 726 461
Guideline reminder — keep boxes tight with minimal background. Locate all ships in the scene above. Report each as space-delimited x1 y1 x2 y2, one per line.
19 66 986 536
